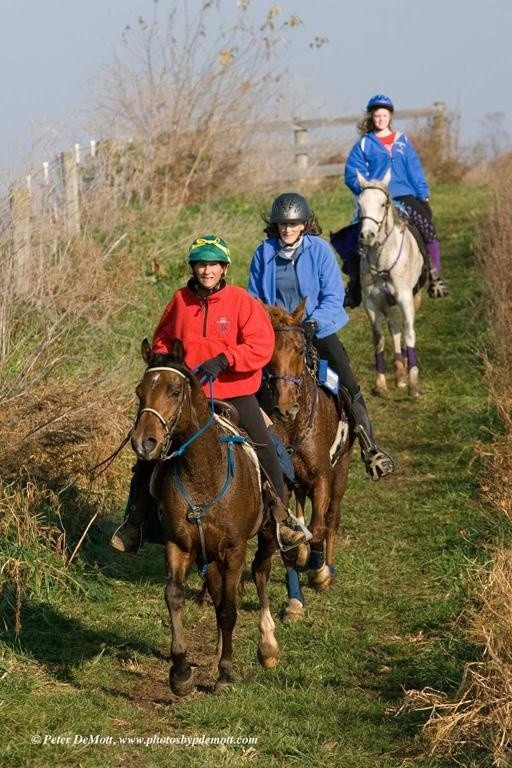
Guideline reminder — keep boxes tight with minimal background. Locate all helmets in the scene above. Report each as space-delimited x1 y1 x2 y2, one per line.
367 95 395 112
271 193 309 224
188 235 231 263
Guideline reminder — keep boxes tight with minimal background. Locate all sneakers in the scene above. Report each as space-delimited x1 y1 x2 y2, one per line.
344 288 362 308
428 278 449 298
280 521 305 546
110 522 142 552
360 445 395 481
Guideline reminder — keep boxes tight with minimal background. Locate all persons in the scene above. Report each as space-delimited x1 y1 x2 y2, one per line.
241 192 393 484
339 95 448 309
107 231 312 554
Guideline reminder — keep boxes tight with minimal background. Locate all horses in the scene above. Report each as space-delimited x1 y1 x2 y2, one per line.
354 167 429 398
254 295 356 628
131 337 292 698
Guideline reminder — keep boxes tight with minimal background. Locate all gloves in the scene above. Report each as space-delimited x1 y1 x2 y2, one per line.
304 322 317 337
151 352 166 366
200 352 228 381
